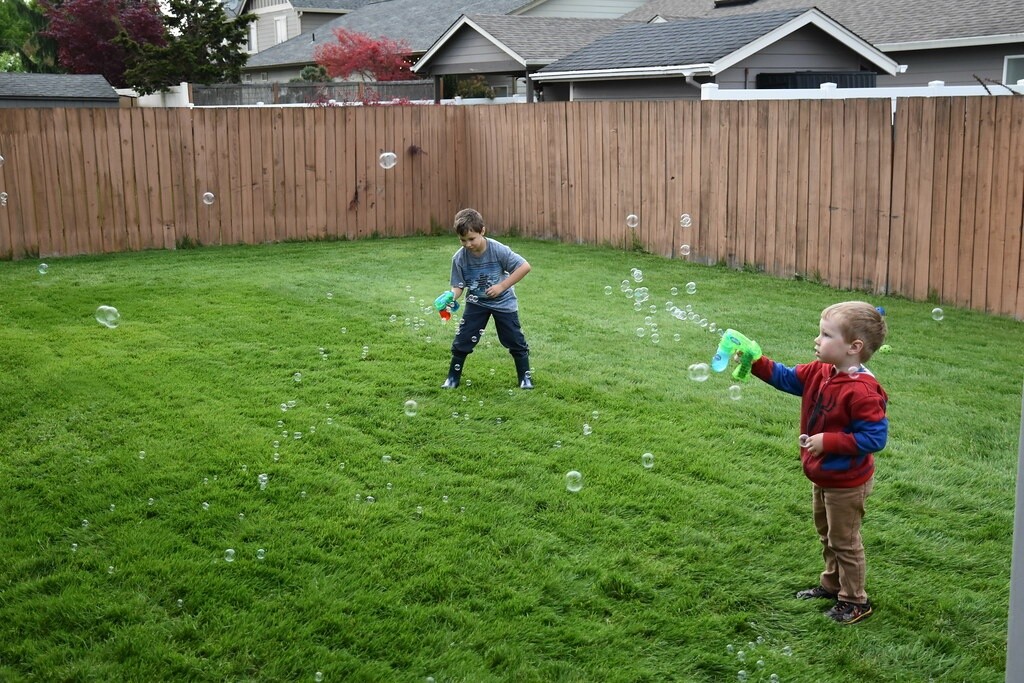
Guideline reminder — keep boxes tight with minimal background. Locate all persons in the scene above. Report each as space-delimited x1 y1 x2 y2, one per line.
441 208 539 390
735 301 888 623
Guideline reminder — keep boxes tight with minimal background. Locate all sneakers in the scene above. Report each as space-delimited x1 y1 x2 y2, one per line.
796 587 839 601
823 600 873 624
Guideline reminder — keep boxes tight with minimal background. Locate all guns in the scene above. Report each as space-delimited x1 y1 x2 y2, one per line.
712 328 763 381
433 291 460 321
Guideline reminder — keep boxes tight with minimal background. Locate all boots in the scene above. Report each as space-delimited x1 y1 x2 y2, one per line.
441 356 465 390
515 356 534 388
